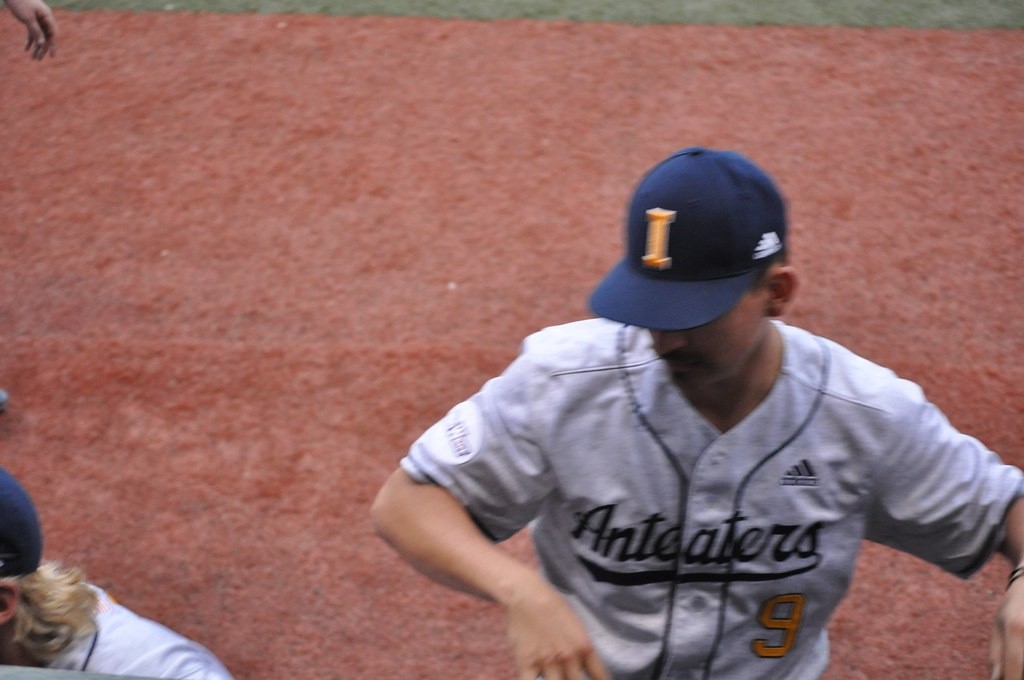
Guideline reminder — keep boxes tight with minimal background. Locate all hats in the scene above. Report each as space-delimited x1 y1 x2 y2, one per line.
0 467 42 578
589 146 787 333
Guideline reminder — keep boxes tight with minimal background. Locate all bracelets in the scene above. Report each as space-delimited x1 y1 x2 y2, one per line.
1005 567 1024 589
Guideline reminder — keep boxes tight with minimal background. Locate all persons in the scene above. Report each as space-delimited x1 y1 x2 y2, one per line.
0 0 59 412
371 147 1024 680
0 467 233 680
